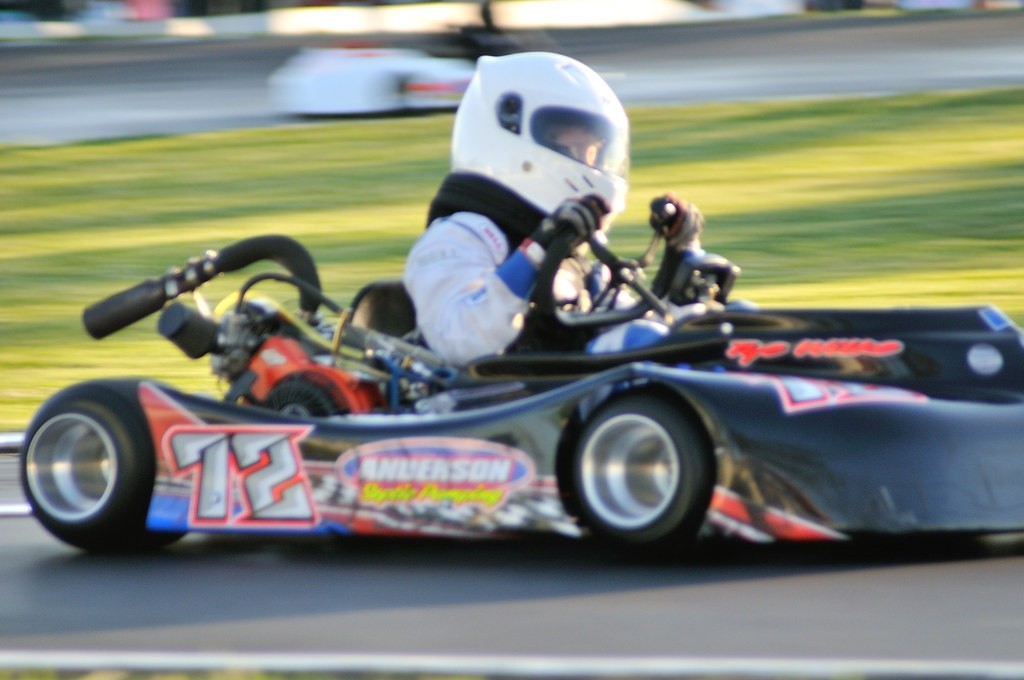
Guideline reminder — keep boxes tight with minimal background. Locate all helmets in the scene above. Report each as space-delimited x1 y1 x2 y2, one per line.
446 49 629 242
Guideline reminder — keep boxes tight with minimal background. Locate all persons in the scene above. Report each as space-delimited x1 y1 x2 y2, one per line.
402 51 705 366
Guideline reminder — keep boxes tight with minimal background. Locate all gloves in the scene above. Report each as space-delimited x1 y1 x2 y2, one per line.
527 195 611 250
647 194 702 250
